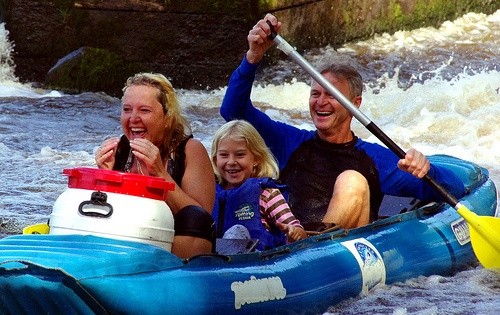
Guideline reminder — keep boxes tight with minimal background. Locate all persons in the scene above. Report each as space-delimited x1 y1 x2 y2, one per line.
209 118 309 256
218 12 468 236
94 72 218 262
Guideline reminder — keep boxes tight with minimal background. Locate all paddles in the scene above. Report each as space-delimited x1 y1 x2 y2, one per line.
264 20 500 270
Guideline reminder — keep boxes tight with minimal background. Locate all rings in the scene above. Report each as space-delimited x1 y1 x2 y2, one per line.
150 143 154 148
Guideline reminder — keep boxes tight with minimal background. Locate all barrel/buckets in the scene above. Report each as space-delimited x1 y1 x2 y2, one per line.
49 166 177 253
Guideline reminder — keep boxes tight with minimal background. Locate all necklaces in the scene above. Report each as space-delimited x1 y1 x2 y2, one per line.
135 156 146 175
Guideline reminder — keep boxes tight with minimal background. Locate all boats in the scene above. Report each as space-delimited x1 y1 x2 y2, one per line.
0 152 499 315
